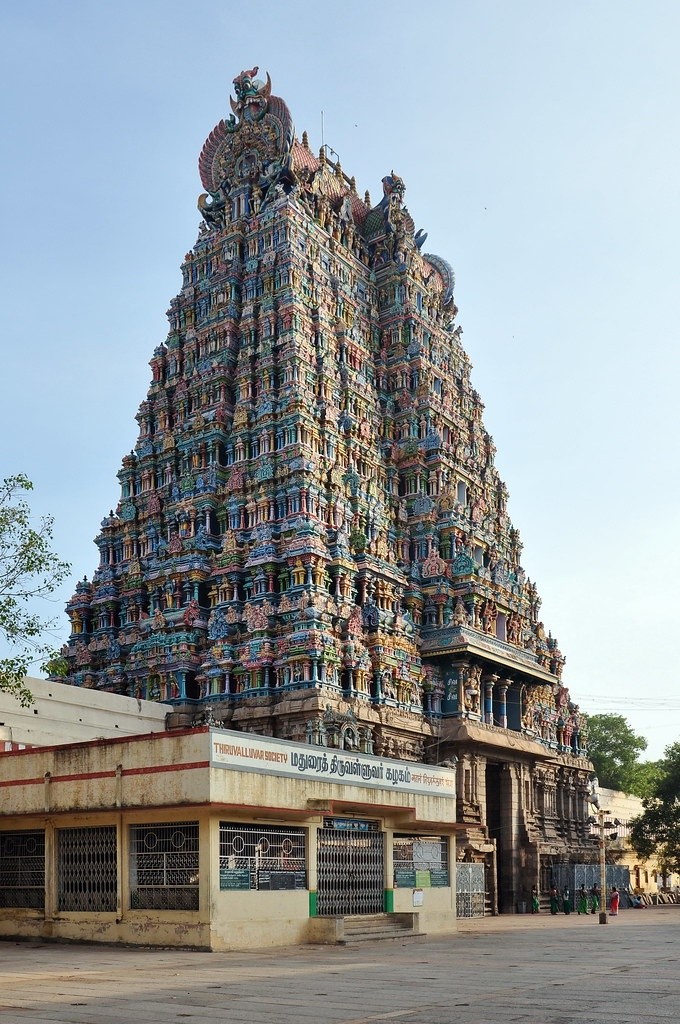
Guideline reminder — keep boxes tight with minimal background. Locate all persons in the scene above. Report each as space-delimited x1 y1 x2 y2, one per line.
49 167 591 751
591 883 601 913
633 896 647 909
562 885 572 915
609 887 619 916
550 885 559 915
578 884 591 915
657 887 666 896
531 885 540 914
674 886 680 904
